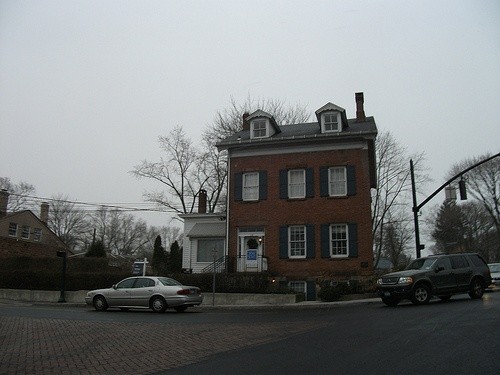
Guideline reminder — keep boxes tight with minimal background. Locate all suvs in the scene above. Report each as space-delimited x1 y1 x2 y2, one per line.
374 252 492 307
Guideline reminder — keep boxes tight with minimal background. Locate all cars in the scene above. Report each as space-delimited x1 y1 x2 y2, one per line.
85 275 202 312
487 263 500 285
